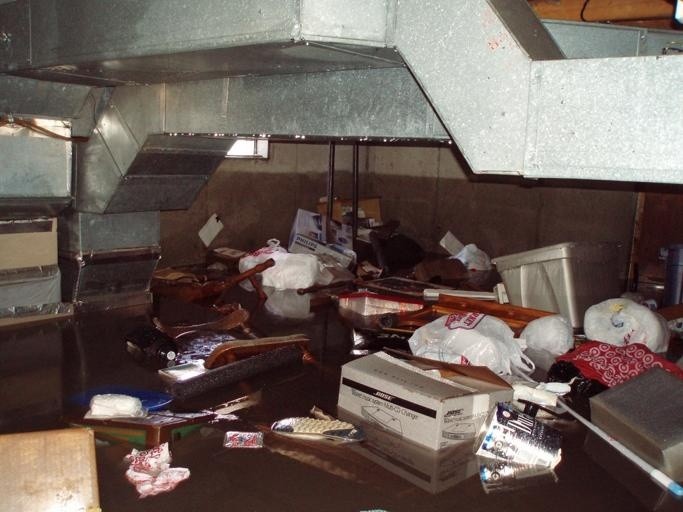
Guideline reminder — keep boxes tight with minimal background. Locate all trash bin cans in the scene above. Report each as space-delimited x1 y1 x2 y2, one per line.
69 387 173 413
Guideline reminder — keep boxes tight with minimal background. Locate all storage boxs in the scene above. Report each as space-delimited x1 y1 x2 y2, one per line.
336 408 480 495
336 350 515 451
492 237 624 333
284 194 383 268
